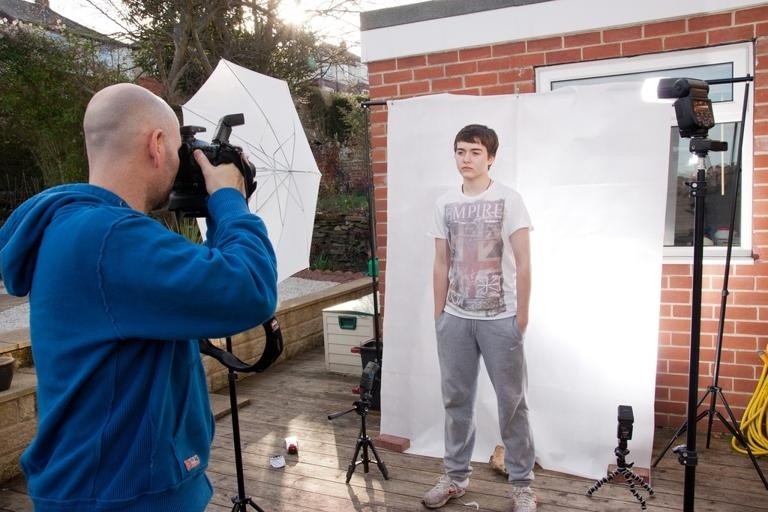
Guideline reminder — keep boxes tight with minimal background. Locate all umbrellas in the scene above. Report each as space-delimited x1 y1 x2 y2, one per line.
178 56 323 285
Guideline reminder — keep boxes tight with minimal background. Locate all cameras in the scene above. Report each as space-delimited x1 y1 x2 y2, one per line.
169 126 257 219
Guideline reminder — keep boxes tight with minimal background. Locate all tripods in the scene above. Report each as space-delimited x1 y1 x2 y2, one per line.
649 156 767 488
345 358 393 484
206 220 264 511
584 406 657 511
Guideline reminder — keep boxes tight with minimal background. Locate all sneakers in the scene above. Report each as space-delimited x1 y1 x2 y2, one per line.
420 475 471 510
511 486 537 512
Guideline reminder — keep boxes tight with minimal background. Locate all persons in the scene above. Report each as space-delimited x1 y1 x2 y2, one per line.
0 83 279 512
422 124 537 511
